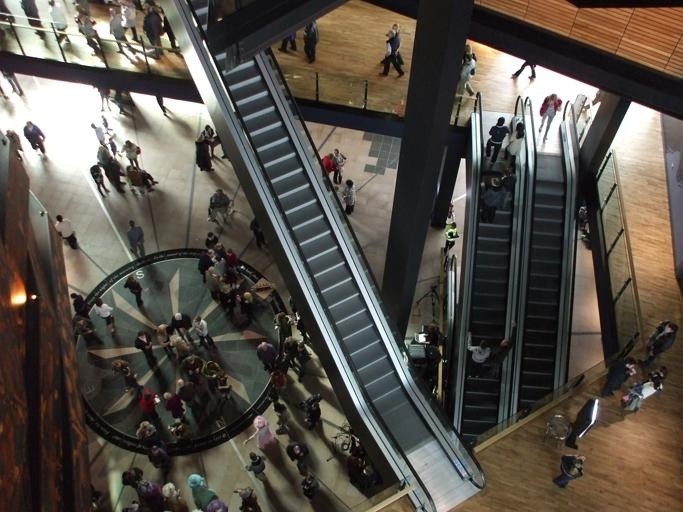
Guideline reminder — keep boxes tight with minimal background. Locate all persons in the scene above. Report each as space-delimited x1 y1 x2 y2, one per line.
567 395 599 450
466 335 490 378
479 115 527 222
302 21 317 63
553 452 585 489
599 318 678 414
45 118 356 511
277 33 298 53
379 22 478 105
6 131 24 161
0 2 178 116
537 94 562 143
426 322 439 350
443 222 457 257
510 56 536 82
22 121 47 159
490 320 516 377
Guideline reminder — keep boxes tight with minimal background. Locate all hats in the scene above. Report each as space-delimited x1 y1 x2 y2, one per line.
187 473 201 488
162 483 176 499
253 416 267 430
